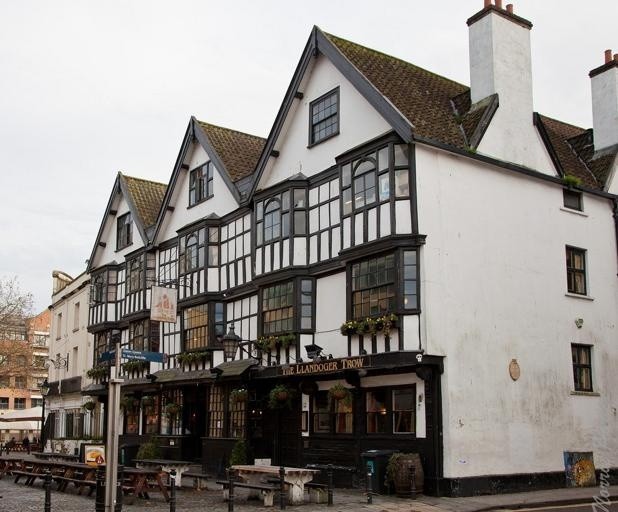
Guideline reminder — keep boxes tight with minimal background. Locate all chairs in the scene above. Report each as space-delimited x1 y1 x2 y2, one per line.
0 453 189 504
215 477 282 512
261 476 335 507
0 436 42 453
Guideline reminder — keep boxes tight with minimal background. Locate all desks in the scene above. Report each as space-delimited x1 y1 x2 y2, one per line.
226 460 320 504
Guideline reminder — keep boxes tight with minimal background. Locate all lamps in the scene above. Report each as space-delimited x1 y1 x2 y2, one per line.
221 322 245 362
296 339 334 363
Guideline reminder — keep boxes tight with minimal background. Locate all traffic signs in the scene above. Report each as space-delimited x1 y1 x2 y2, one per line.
122 350 167 362
97 350 115 362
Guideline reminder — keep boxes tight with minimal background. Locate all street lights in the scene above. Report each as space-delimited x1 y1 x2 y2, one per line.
38 378 52 445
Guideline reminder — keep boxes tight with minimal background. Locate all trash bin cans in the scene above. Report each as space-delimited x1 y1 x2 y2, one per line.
360 449 400 495
120 443 140 467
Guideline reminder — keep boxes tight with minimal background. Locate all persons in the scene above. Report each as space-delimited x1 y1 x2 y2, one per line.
6 437 17 447
22 435 30 447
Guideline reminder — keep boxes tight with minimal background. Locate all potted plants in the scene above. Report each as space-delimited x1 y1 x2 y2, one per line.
80 399 96 416
121 391 183 422
231 381 353 406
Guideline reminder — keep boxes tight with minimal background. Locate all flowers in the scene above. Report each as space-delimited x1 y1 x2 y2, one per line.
253 333 297 353
340 313 400 336
